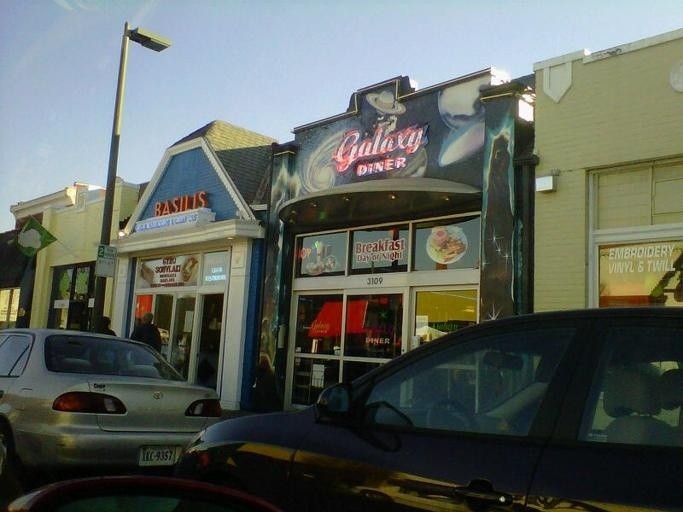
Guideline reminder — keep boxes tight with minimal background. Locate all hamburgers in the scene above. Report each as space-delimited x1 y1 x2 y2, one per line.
429 229 450 251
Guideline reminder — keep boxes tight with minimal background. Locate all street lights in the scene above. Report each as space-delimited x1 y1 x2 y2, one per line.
90 21 172 332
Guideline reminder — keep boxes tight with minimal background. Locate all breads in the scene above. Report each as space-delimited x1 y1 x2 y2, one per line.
183 258 197 283
141 264 154 283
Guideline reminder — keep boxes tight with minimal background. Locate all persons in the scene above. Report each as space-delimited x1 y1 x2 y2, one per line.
130 314 161 354
104 317 116 336
486 352 562 433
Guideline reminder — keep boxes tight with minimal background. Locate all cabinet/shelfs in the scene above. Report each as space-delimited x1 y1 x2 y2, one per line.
291 356 328 406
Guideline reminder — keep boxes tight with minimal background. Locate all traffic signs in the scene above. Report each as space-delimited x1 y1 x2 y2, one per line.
94 243 117 279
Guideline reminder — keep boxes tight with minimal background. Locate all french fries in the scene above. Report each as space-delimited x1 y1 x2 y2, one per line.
446 240 465 262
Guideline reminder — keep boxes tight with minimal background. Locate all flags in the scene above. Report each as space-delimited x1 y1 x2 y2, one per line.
7 218 58 258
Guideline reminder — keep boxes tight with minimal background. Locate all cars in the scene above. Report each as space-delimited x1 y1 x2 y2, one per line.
150 331 216 377
178 305 681 512
0 327 225 476
